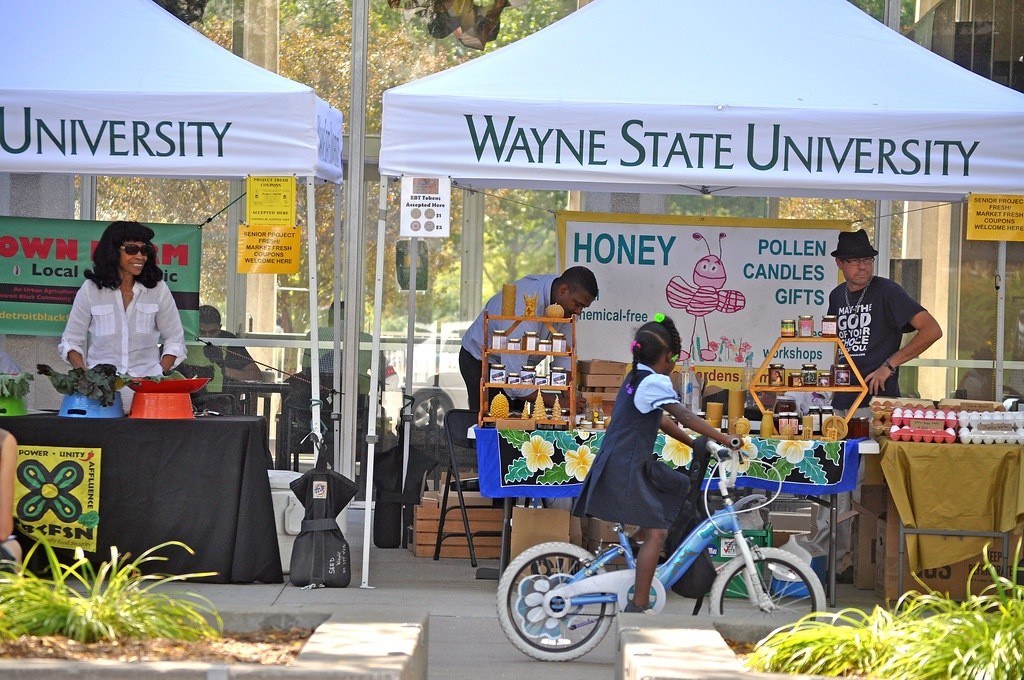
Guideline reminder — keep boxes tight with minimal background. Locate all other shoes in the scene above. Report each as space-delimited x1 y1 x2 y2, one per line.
836 565 854 584
624 600 655 614
620 537 640 559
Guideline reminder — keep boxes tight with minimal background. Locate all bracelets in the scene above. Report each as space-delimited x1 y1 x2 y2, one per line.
883 361 896 375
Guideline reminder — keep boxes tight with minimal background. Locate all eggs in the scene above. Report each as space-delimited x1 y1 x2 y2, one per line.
873 400 1024 437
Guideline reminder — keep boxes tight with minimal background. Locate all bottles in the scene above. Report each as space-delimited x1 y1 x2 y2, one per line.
760 410 774 438
677 359 691 387
740 360 756 409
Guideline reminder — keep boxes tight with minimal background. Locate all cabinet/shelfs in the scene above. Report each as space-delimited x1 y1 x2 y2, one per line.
748 337 869 439
477 310 577 431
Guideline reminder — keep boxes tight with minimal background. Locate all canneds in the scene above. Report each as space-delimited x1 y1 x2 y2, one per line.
798 315 814 337
489 363 568 386
768 363 851 386
772 396 835 435
491 330 567 353
821 314 838 338
780 319 795 338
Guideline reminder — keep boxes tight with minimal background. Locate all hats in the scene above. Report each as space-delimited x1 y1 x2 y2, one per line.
831 229 878 258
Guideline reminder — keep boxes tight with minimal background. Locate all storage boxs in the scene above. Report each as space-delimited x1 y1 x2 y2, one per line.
577 359 627 375
511 507 582 584
768 533 827 597
266 469 304 574
588 538 628 565
578 374 624 388
704 508 773 598
876 490 1024 602
852 484 890 590
589 516 641 543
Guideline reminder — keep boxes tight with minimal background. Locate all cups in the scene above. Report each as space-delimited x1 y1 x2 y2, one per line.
677 373 693 411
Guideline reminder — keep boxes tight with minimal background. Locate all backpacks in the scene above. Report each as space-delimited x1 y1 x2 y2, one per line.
664 436 717 599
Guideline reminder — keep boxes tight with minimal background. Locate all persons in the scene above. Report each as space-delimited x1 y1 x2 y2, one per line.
57 221 188 415
813 229 944 585
172 305 265 404
302 302 386 407
958 347 1020 400
0 428 23 575
458 266 599 412
570 313 745 613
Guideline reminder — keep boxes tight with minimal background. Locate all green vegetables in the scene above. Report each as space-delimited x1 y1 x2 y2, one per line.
0 363 189 408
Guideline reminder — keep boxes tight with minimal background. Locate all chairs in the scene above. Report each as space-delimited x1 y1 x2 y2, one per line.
433 409 512 568
275 368 380 468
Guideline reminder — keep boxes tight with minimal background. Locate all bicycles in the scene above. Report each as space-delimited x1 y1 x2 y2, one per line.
494 438 826 663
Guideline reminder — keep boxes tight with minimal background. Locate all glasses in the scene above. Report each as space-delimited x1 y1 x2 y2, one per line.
120 244 154 256
844 257 875 266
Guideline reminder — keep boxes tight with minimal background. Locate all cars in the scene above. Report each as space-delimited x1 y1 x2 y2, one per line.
400 322 472 428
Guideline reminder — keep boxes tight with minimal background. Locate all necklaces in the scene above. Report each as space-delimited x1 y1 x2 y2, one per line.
845 276 874 314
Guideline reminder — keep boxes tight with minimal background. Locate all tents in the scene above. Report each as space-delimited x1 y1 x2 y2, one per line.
0 0 343 469
359 0 1024 589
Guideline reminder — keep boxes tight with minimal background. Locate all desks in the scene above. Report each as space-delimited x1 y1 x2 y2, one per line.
205 378 292 471
0 409 284 586
856 435 1024 611
467 420 880 608
